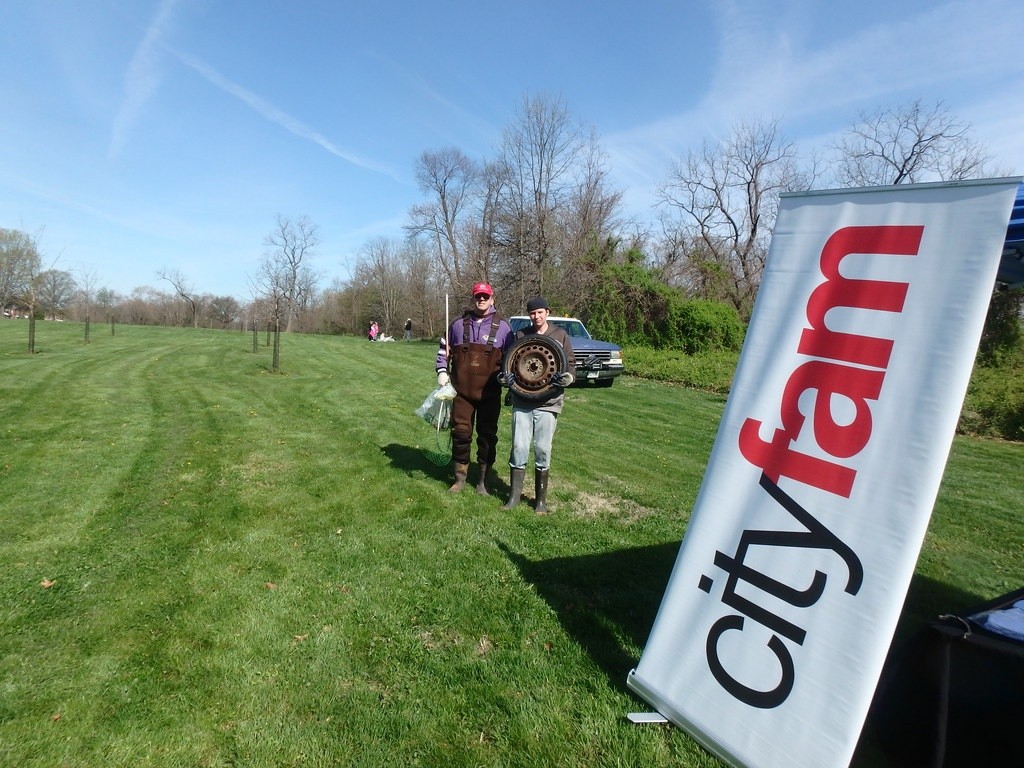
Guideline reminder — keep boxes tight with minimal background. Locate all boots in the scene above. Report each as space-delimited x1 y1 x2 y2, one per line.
448 461 469 492
534 468 549 515
501 467 525 509
477 463 491 497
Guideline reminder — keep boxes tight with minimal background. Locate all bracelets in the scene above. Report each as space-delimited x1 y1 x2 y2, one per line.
437 368 448 374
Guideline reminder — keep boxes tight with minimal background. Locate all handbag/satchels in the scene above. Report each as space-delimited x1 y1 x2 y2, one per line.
416 384 457 429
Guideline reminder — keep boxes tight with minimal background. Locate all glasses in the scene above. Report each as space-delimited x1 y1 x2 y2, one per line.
474 293 490 300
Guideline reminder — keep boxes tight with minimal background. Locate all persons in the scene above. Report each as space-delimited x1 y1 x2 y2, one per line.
368 320 379 341
403 318 411 343
496 297 575 517
436 282 515 497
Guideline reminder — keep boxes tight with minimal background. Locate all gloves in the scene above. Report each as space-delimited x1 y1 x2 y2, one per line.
496 372 515 387
553 371 573 387
438 372 450 386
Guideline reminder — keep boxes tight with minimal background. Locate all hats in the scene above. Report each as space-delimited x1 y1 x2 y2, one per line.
473 283 493 297
527 298 553 315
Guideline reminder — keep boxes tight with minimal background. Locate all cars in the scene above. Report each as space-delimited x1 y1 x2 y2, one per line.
4 308 63 322
508 315 625 388
